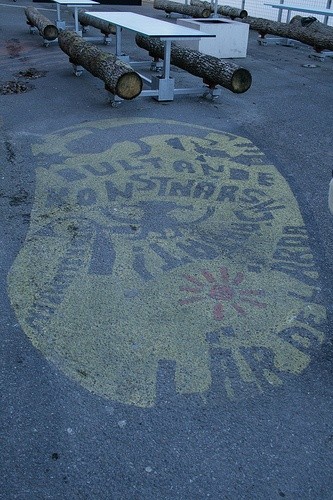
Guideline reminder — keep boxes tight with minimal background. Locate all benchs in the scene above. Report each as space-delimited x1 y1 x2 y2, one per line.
75 8 122 45
136 32 252 96
24 6 58 47
58 29 142 106
153 0 209 18
190 0 248 21
241 16 333 52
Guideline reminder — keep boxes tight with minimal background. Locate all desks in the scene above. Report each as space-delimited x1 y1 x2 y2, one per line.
55 0 100 35
85 12 216 102
273 4 333 46
